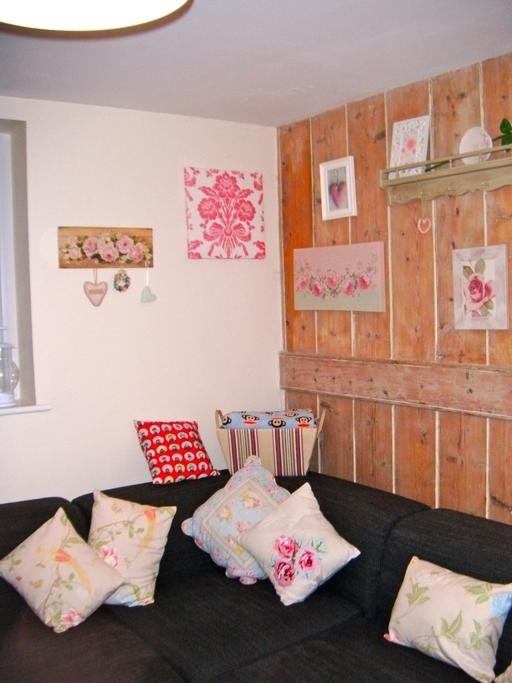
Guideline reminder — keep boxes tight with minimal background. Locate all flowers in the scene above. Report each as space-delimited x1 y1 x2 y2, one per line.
60 229 153 267
294 252 378 301
461 259 498 319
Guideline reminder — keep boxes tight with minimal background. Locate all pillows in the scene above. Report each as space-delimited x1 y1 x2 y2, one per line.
133 420 221 484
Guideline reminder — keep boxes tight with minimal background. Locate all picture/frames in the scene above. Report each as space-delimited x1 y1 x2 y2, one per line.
387 114 430 183
319 156 357 221
450 244 510 331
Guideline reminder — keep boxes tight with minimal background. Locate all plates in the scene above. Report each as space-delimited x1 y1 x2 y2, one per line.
458 127 492 165
